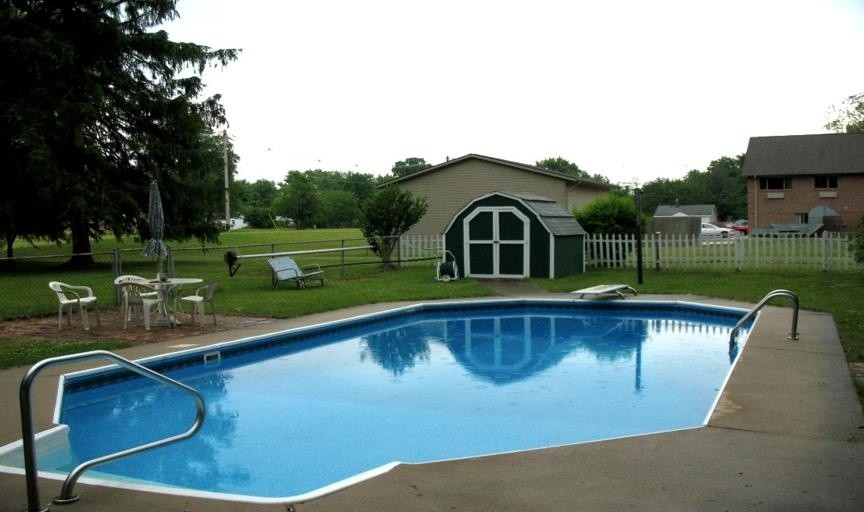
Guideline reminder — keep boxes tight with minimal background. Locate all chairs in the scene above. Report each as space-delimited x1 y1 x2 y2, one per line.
49 275 219 332
267 255 326 289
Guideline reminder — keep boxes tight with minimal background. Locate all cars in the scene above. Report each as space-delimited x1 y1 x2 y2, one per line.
701 221 749 239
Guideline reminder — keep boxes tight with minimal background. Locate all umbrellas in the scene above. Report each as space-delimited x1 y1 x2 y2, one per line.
142 180 167 317
